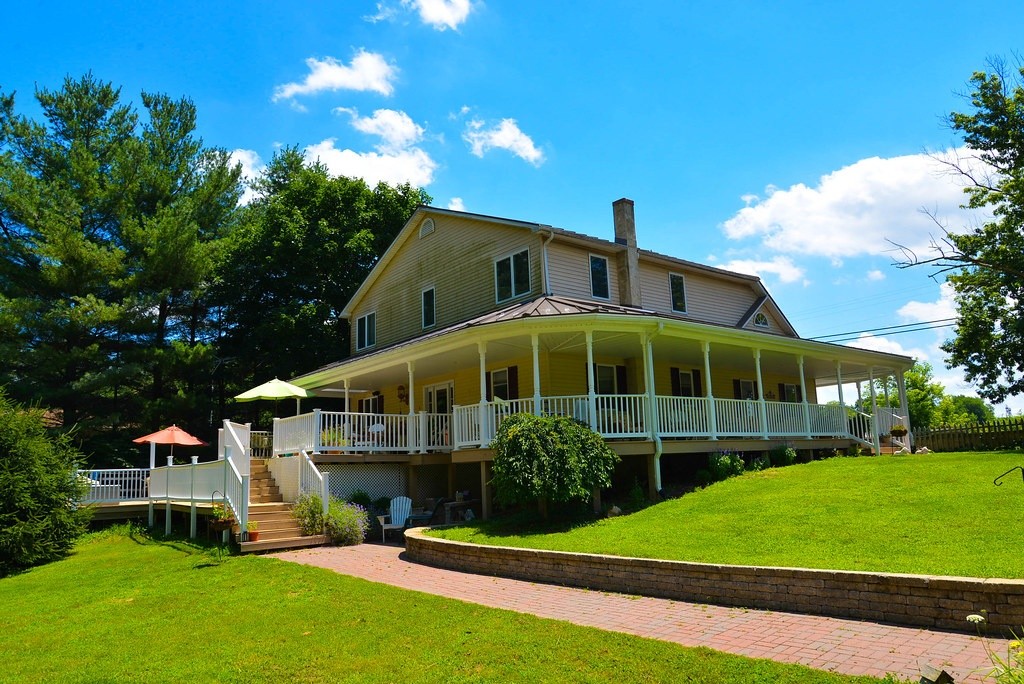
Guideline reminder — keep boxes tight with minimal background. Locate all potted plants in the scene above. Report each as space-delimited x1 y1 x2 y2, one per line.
879 433 892 443
890 424 907 437
206 503 236 528
247 521 260 542
321 427 350 456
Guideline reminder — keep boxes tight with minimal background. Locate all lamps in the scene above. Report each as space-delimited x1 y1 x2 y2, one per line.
396 386 409 406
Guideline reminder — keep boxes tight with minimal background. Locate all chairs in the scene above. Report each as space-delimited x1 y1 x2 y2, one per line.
406 497 443 528
376 497 414 544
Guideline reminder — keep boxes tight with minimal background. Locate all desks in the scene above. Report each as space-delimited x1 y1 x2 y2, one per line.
444 499 482 524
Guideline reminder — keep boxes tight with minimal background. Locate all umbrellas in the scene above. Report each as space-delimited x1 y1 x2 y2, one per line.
233 375 318 417
132 424 210 456
